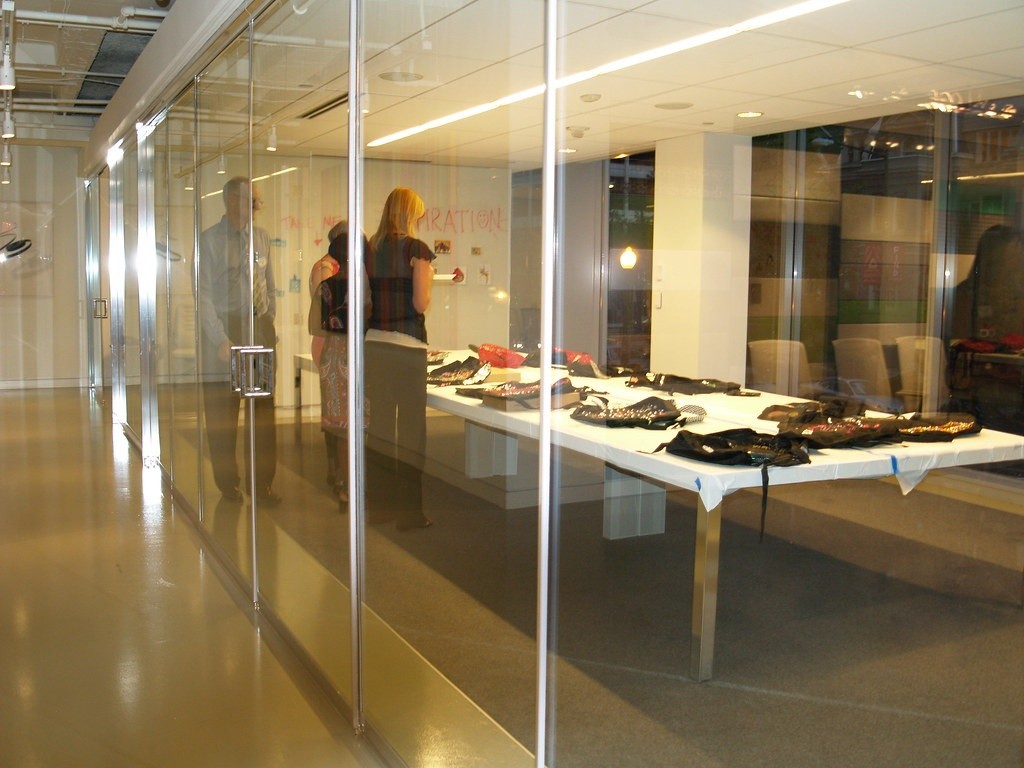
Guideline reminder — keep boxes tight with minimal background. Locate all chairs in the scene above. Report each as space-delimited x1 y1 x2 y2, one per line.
749 341 815 403
824 337 897 420
896 335 953 415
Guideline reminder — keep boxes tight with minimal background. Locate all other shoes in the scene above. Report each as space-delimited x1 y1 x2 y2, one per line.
328 474 350 511
222 489 244 503
396 518 432 530
256 490 284 506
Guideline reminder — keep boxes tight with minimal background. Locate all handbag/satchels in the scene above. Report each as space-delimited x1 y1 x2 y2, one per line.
427 356 491 387
456 377 576 400
522 345 639 379
479 343 527 368
427 352 447 366
758 402 979 452
667 429 811 468
624 372 743 395
569 396 681 427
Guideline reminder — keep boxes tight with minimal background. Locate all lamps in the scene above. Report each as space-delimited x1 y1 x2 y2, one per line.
1 137 12 166
0 45 16 91
1 165 11 185
2 95 15 139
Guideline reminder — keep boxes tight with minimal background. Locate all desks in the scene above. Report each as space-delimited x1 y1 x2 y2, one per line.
293 349 1024 682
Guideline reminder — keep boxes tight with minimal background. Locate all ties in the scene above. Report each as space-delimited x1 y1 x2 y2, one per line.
236 230 262 319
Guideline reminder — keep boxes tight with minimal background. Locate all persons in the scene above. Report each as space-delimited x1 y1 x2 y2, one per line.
309 218 366 486
308 238 371 516
188 175 284 508
363 186 437 534
941 222 1024 434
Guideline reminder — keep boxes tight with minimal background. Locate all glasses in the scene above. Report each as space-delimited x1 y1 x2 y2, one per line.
233 194 263 209
